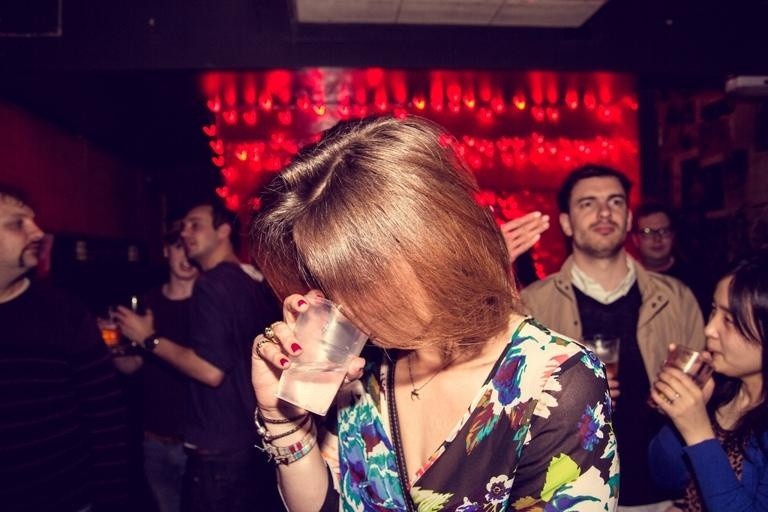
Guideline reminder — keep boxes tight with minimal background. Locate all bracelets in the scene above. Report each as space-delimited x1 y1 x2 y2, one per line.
254 404 312 423
261 430 321 463
251 416 314 439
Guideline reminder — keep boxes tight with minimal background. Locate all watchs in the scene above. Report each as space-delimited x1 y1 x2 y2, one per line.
142 332 160 353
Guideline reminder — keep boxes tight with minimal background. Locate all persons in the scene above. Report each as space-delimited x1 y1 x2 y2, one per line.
92 220 237 511
109 202 283 507
501 210 552 265
638 259 767 512
250 109 624 512
0 186 131 508
516 163 710 512
630 204 714 320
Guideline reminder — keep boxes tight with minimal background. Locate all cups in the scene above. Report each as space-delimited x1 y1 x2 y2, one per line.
579 334 620 413
647 344 716 416
96 311 123 350
274 295 373 420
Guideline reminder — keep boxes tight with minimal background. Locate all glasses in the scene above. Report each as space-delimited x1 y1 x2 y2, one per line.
636 227 674 239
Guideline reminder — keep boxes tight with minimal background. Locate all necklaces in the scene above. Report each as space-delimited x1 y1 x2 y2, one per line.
406 351 467 400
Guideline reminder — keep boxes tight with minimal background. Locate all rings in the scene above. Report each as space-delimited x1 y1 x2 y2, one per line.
255 339 271 358
671 392 680 401
264 322 284 344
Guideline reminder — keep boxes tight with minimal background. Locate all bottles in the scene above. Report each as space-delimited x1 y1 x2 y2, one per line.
127 293 145 349
